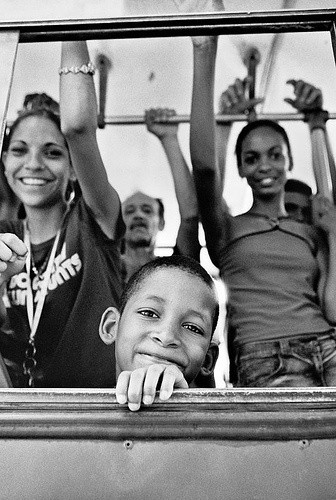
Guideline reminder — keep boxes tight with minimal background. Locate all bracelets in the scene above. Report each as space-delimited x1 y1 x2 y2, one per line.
58 62 94 75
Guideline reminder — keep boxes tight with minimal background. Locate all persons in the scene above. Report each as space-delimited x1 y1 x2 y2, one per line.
0 0 336 388
99 255 221 412
